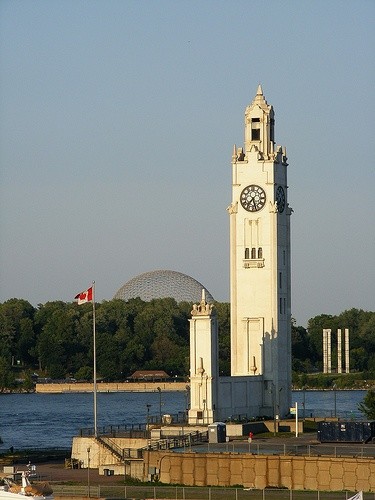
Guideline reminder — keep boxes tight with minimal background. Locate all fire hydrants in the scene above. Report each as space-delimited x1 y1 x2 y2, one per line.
249 432 253 439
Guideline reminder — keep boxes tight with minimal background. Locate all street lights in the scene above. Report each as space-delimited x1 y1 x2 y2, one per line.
87 447 90 499
303 386 308 417
145 405 151 437
332 383 337 418
157 386 162 421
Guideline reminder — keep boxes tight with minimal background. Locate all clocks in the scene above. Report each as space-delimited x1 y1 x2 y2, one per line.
276 186 286 213
240 185 266 212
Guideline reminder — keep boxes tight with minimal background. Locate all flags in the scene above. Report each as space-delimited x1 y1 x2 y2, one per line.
74 286 92 305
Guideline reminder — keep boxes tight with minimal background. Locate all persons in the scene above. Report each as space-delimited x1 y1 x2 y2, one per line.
249 432 253 444
